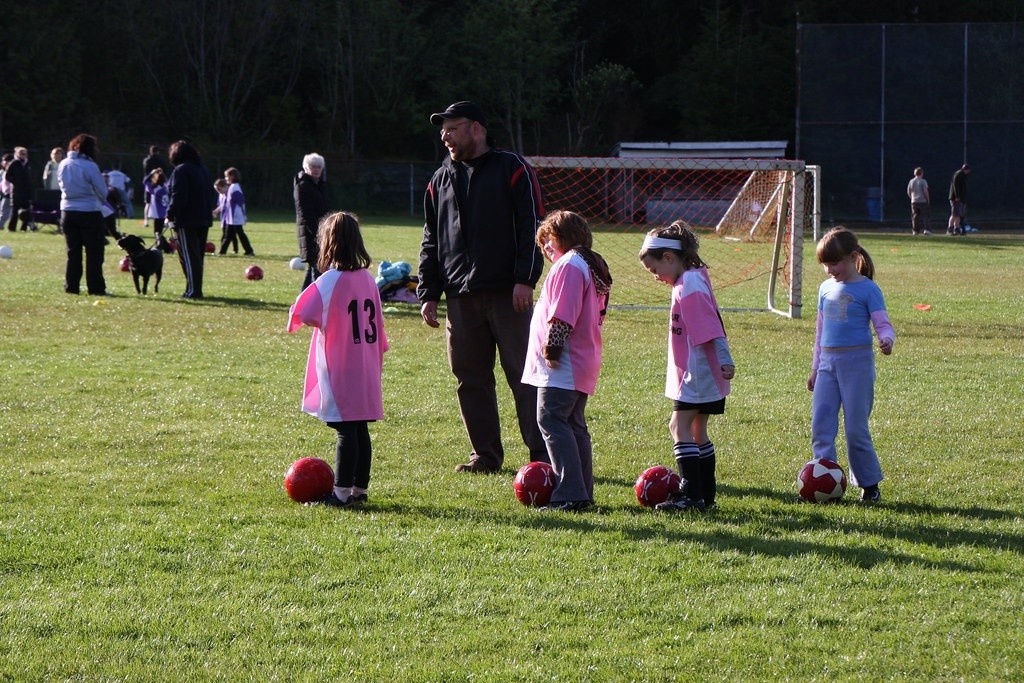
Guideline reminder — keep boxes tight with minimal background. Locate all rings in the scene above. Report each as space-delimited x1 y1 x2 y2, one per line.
524 301 529 304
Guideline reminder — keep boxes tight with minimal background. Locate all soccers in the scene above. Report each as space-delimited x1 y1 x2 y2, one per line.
205 242 215 253
289 257 306 270
636 466 681 507
285 457 334 502
0 246 13 259
513 461 556 508
119 258 130 272
797 459 848 503
245 265 263 281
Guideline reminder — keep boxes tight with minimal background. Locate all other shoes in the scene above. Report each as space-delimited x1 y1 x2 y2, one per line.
326 492 356 509
455 451 498 475
924 230 932 236
353 493 368 502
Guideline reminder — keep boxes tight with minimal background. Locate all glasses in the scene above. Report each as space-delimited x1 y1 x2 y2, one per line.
440 121 471 135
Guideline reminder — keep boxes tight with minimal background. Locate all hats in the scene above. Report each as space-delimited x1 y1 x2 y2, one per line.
430 101 489 130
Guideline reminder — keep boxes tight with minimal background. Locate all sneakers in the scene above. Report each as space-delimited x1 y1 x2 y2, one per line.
656 477 705 512
860 489 880 505
549 501 592 512
704 500 717 512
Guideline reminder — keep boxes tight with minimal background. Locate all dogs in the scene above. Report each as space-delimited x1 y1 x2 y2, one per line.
155 232 173 253
118 234 163 294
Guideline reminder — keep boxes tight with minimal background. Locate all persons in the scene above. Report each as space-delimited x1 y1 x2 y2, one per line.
294 154 326 293
806 225 895 507
520 211 614 512
415 101 545 471
639 220 735 512
287 212 391 510
908 168 933 236
0 133 255 298
946 164 971 236
